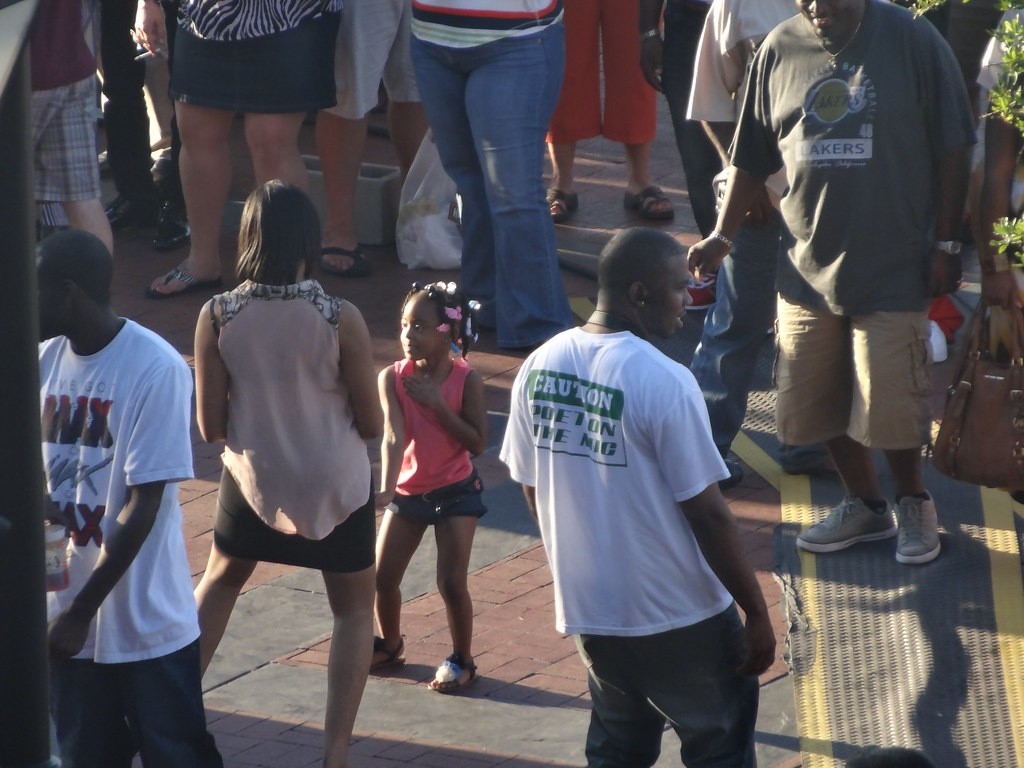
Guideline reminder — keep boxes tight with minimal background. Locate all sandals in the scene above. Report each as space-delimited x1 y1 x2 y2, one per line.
372 636 405 668
428 656 478 691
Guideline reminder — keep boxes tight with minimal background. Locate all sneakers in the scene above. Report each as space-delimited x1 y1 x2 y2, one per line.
893 490 940 563
796 496 896 552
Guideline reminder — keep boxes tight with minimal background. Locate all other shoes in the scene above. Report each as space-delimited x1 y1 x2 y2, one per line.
718 459 743 490
686 270 717 309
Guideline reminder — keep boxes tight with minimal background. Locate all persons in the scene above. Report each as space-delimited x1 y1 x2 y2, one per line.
36 230 222 768
499 227 777 768
375 282 489 692
546 0 1024 565
28 0 575 355
194 179 383 768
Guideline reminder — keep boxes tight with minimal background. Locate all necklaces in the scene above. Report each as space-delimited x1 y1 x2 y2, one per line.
812 21 863 70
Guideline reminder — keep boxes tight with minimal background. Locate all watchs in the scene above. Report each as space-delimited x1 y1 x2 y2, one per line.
935 240 964 256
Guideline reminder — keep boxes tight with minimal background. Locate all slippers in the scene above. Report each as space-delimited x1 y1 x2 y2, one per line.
623 185 674 218
319 247 372 276
146 263 222 298
546 189 578 223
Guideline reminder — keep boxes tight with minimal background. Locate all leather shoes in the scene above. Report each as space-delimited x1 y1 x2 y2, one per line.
152 199 191 248
104 194 152 228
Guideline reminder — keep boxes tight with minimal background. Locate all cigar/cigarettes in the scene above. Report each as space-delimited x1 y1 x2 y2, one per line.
135 48 160 61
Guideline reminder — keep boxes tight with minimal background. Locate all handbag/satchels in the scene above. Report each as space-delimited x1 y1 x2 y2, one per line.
931 293 1024 491
395 127 464 270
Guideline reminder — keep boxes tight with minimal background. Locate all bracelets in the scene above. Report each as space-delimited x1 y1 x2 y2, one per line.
979 252 1010 273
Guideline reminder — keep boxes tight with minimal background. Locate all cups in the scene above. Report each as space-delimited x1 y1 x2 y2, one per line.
45 524 69 591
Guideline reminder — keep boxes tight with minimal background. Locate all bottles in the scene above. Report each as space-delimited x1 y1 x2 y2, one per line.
929 321 948 362
